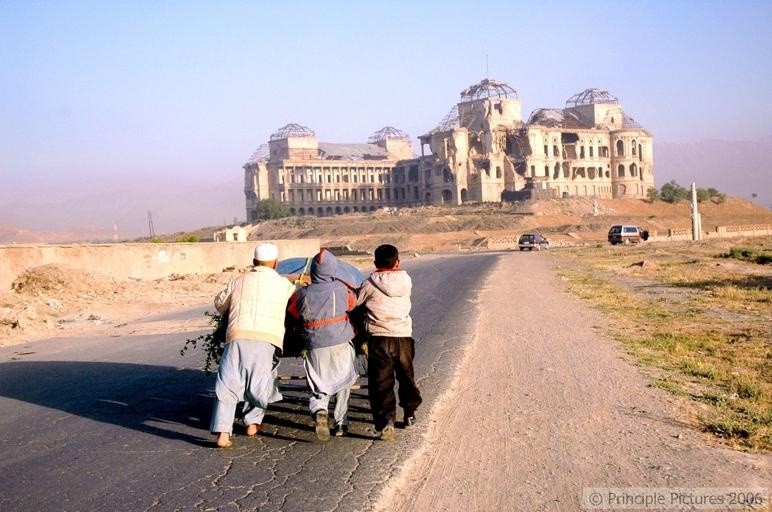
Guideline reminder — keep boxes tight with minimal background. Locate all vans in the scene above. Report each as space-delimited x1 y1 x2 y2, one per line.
607 224 650 245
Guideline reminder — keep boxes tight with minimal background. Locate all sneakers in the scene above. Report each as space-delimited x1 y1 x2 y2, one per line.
216 438 232 448
313 408 332 442
380 420 396 441
246 422 262 437
405 416 418 429
334 425 349 437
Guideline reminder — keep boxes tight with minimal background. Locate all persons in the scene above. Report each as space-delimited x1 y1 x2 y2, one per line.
356 244 423 442
284 248 358 443
210 243 297 448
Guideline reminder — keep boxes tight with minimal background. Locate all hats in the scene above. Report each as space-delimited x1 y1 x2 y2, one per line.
253 243 278 263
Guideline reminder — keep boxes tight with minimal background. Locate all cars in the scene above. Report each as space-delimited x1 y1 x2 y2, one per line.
517 233 550 251
